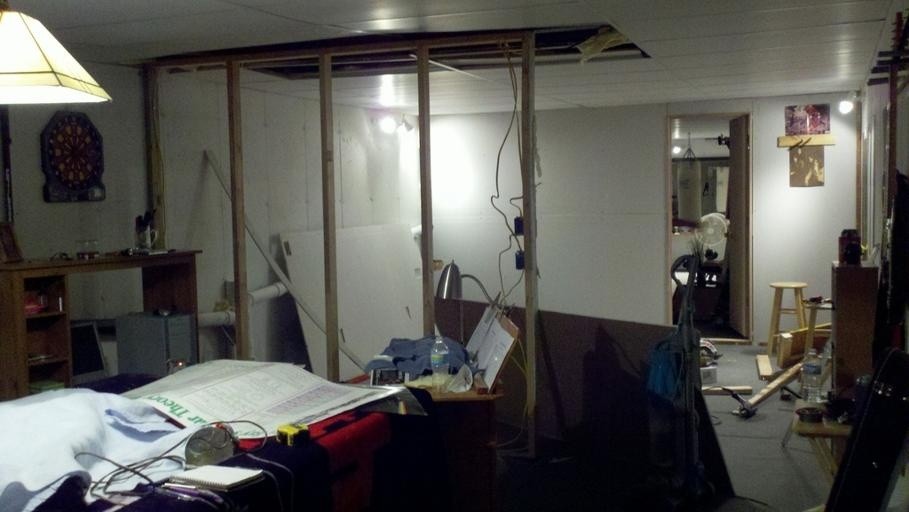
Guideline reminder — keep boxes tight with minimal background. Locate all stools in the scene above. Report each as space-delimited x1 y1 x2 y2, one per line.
767 282 808 358
797 399 855 487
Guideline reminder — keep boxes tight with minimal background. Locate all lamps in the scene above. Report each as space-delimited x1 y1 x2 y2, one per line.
435 261 502 317
0 11 112 262
839 91 857 115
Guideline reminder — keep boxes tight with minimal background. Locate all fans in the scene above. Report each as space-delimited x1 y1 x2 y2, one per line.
695 214 726 264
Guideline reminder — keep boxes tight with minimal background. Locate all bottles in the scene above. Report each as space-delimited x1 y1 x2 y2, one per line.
802 349 821 402
431 336 449 392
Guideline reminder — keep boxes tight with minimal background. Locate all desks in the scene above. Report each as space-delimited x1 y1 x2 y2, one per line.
378 373 503 460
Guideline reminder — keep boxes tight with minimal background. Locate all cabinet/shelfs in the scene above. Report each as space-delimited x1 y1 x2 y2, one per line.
3 247 202 404
830 260 878 407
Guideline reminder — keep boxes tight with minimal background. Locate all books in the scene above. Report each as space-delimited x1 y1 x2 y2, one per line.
167 464 268 495
